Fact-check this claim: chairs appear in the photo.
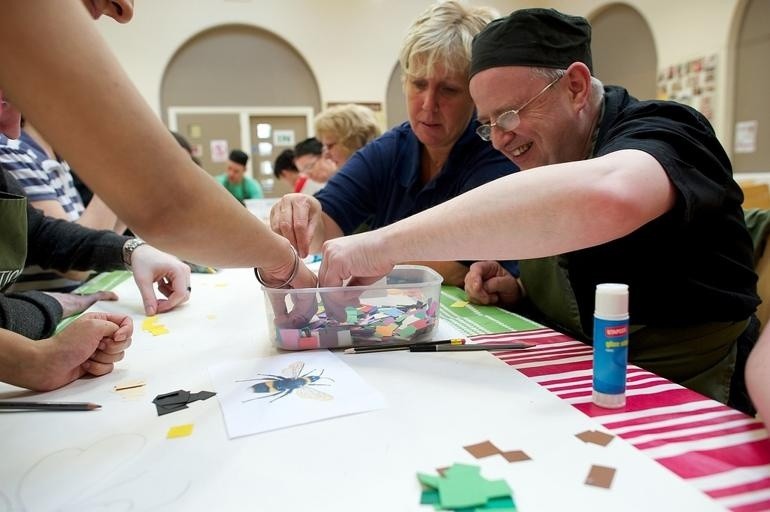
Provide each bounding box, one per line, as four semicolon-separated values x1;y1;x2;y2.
741;208;766;334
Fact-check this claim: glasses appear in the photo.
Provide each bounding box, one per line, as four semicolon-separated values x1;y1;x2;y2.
475;75;562;142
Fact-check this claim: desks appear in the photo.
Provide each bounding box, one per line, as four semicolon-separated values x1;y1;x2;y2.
0;200;770;512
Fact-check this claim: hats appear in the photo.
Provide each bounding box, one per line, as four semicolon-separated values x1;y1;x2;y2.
229;151;249;165
467;8;594;86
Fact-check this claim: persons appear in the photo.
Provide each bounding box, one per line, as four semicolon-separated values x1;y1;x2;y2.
1;1;320;392
1;99;202;340
273;103;387;196
317;7;761;420
213;149;265;200
745;320;770;432
270;1;522;286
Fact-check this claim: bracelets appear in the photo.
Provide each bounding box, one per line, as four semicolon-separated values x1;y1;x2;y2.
254;246;299;287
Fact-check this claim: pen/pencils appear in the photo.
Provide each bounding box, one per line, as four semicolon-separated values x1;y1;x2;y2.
343;339;466;353
409;343;536;352
0;401;103;410
